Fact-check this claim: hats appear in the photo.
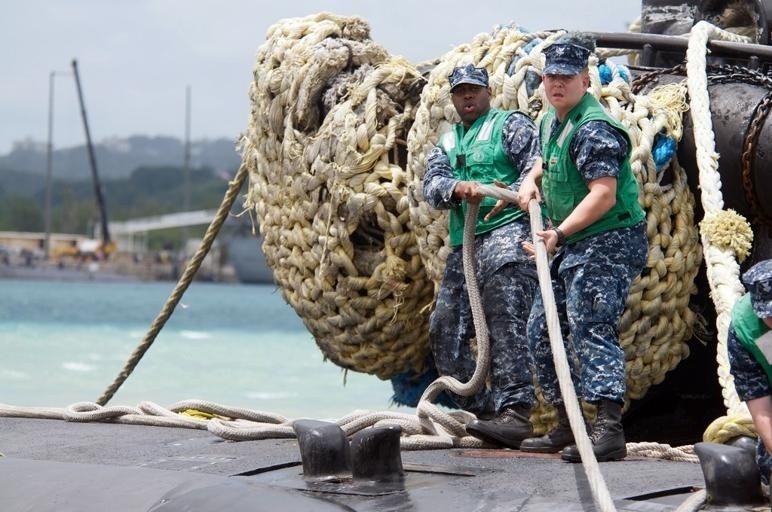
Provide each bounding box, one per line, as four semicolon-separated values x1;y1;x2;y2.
541;43;589;76
448;66;488;92
743;259;771;319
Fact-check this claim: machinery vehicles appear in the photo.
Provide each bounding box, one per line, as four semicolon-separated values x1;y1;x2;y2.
51;57;120;268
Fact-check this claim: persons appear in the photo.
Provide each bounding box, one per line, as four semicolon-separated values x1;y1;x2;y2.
727;258;771;490
518;39;652;463
421;62;543;450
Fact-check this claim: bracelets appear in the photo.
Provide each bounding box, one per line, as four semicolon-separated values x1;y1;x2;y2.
553;227;565;249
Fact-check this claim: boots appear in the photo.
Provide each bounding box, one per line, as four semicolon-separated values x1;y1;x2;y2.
466;407;532;449
521;401;592;453
562;399;627;460
